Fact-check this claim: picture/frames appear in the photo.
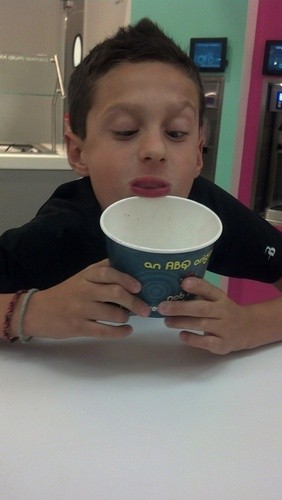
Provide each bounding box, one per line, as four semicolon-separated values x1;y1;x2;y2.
190;37;227;72
262;37;282;76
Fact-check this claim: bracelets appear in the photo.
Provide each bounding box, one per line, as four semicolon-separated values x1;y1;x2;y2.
3;287;37;344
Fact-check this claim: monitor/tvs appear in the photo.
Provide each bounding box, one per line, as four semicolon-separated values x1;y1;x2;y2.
262;40;282;75
190;37;228;72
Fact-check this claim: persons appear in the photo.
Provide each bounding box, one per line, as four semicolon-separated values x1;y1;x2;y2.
0;17;282;355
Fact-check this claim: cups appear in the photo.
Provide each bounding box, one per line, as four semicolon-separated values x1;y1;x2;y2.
100;195;223;319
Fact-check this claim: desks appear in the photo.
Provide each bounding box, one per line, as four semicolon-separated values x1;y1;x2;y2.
0;315;282;500
